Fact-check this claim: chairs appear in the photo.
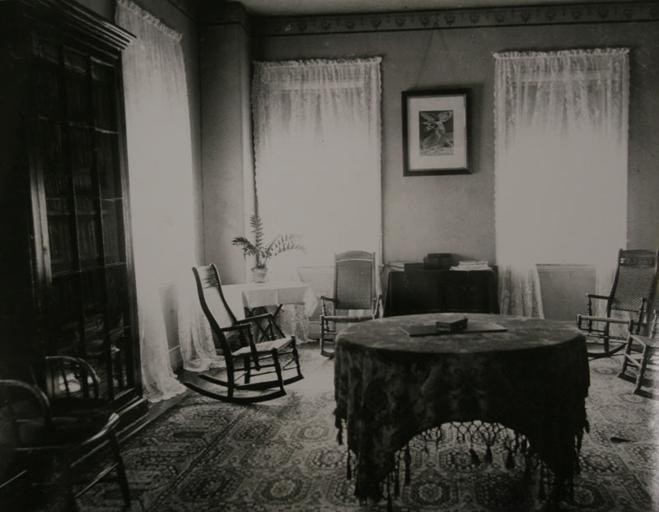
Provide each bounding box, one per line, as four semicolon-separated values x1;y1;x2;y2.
576;249;659;362
1;349;131;511
192;263;304;401
320;250;382;357
617;309;659;393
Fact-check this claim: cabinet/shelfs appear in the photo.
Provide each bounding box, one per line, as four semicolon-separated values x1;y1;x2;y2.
0;0;150;467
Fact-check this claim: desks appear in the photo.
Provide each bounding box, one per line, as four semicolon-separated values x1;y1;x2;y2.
383;264;500;314
203;282;310;344
334;312;591;512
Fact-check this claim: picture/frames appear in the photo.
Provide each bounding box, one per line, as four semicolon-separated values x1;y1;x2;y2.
401;88;472;176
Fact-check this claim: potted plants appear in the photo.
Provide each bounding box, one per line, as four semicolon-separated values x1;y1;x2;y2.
230;215;304;283
